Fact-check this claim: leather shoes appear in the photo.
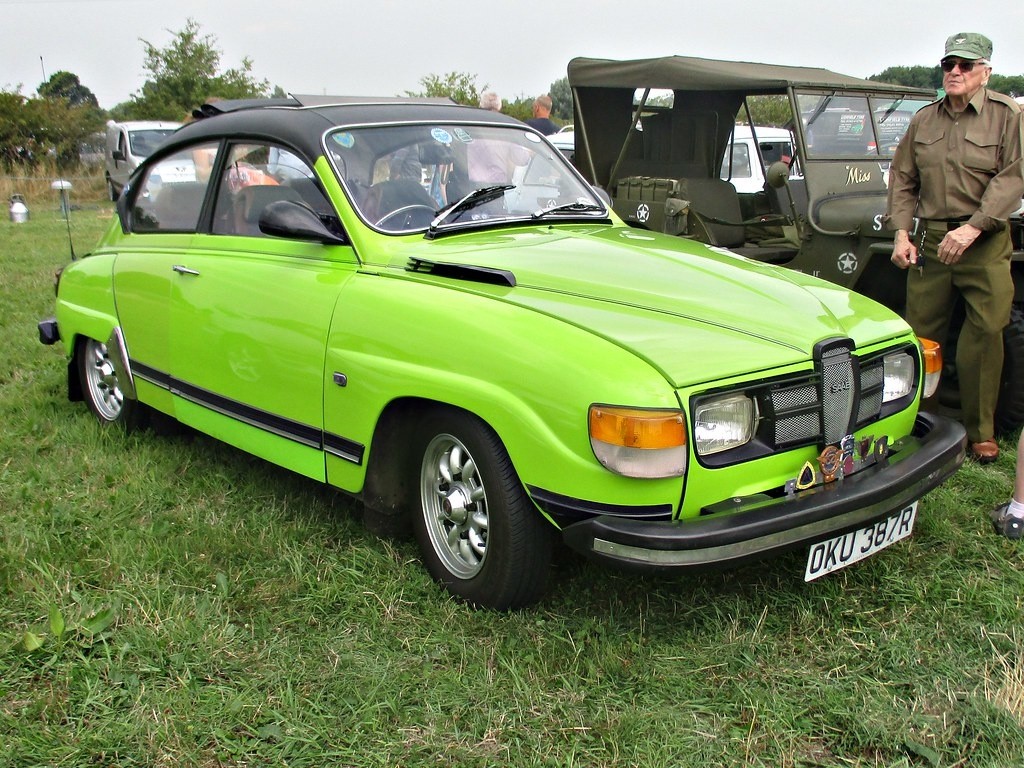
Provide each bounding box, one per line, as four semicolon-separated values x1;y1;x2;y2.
971;435;999;463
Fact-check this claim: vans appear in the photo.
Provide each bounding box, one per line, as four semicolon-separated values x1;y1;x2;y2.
718;124;801;193
104;122;199;207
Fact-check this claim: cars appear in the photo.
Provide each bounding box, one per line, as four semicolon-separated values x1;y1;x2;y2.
546;124;575;151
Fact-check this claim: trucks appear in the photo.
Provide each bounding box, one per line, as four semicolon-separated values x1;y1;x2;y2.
867;101;933;156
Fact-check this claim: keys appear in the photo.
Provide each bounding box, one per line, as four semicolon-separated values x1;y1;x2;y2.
916;255;924;278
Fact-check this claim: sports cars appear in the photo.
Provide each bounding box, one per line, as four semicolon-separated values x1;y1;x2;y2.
39;95;969;614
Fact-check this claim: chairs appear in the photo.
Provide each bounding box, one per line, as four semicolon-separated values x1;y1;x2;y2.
153;168;444;240
679;175;807;263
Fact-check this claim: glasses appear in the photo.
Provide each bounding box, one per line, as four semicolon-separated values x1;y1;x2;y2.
941;61;986;73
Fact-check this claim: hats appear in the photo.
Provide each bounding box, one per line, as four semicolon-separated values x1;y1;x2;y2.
940;33;993;61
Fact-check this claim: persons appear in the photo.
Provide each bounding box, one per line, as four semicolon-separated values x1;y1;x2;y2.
522;97;560;136
267;146;316;184
390;140;421;186
886;31;1024;462
467;89;529;214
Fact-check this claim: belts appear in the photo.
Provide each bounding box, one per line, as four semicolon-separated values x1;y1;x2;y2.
920;217;969;232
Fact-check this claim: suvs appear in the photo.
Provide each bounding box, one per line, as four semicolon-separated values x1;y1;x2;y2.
564;54;1024;438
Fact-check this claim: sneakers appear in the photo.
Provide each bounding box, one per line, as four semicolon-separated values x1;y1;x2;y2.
990;503;1023;540
